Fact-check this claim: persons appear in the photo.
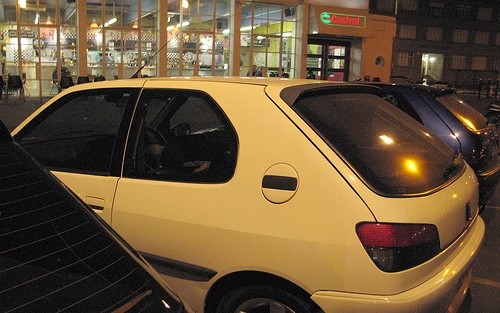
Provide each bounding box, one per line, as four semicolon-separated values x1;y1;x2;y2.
247;65;256;76
307;71;315;79
53;65;57;82
254;65;264;76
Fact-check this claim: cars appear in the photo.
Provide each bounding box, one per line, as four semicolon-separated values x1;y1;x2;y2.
0;120;185;313
9;76;485;313
356;80;499;186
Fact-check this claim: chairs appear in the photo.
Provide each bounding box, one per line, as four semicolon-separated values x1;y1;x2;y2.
61;77;73;88
78;76;92;84
6;76;25;102
